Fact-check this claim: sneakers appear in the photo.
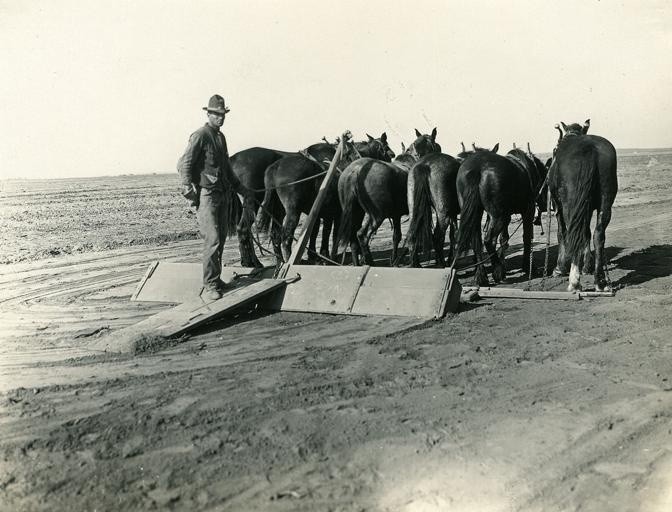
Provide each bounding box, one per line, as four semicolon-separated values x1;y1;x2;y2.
203;279;227;300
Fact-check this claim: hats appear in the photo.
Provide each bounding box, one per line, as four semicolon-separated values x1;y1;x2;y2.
203;95;230;114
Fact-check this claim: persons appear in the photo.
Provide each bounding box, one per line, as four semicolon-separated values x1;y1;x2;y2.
177;94;247;300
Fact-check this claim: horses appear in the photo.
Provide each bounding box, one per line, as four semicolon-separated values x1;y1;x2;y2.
454;143;554;286
220;137;351;267
338;128;441;266
391;143;501;267
254;132;395;265
547;119;619;293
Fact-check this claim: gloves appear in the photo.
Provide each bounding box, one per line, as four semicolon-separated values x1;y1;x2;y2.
183;190;199;214
